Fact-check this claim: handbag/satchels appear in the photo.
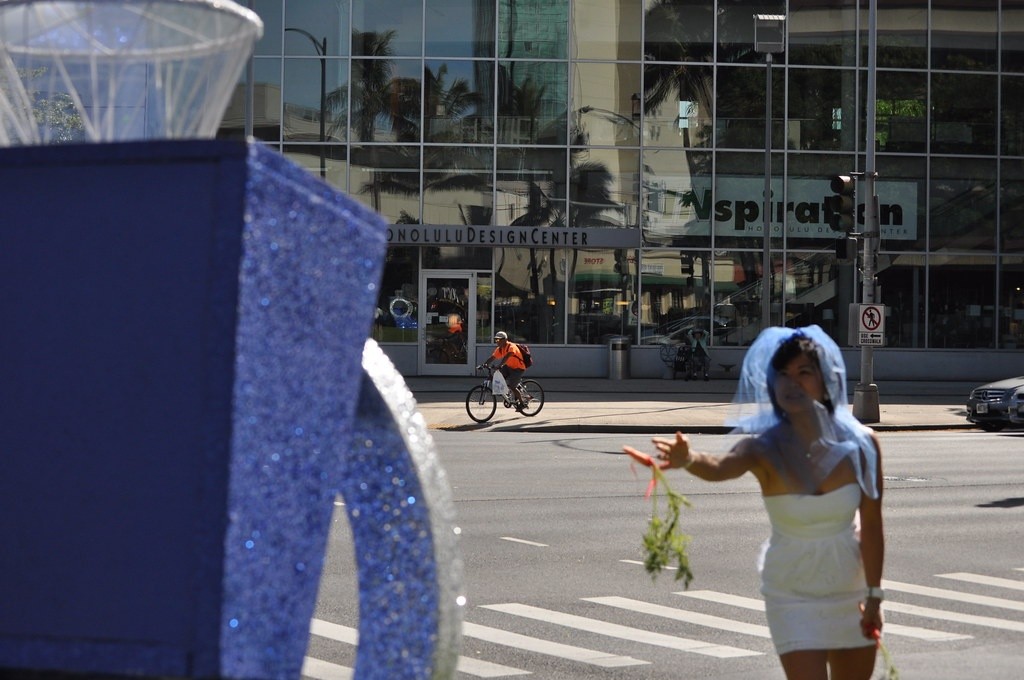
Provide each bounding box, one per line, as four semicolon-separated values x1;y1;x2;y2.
492;369;508;394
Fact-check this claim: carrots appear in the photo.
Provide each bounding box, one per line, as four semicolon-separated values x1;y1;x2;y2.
858;599;886;641
621;445;656;467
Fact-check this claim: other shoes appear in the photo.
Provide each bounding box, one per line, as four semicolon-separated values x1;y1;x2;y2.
693;376;697;380
515;402;526;412
705;376;709;381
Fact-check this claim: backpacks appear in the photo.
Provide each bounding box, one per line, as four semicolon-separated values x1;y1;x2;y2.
499;343;533;368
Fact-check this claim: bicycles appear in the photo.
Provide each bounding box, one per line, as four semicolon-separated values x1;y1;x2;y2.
466;362;544;423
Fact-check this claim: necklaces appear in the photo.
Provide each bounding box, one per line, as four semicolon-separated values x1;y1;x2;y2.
795;439;819;459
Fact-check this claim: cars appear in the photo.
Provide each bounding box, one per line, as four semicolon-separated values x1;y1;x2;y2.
966;375;1024;434
639;314;733;344
427;298;466;325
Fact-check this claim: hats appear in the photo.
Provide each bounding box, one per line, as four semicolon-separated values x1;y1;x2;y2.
493;332;508;340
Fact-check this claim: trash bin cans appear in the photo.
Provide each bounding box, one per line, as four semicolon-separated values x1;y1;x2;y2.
608;337;631;379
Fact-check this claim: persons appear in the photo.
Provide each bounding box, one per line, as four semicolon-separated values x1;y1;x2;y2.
446;306;465;356
652;325;885;680
482;332;527;412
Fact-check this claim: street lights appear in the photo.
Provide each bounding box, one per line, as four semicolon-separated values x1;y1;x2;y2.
284;27;328;180
755;13;787;328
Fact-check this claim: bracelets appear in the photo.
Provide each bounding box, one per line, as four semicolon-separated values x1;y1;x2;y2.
865;587;884;601
685;454;695;470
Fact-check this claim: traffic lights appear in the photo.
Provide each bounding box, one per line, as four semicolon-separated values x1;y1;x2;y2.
829;174;856;232
